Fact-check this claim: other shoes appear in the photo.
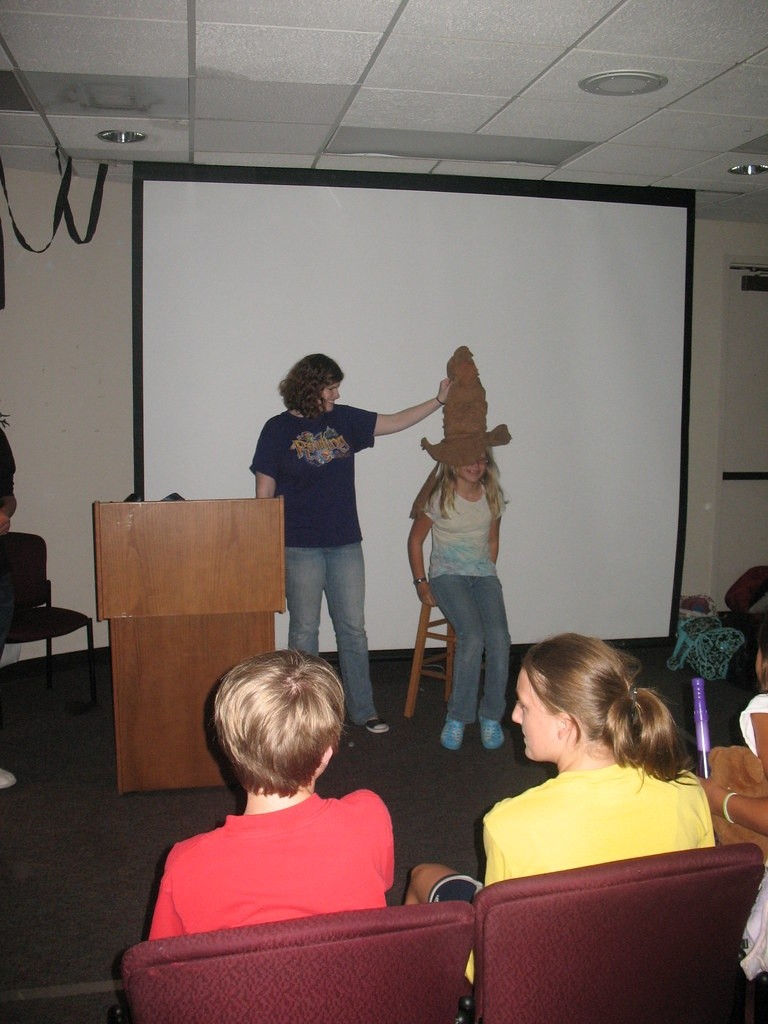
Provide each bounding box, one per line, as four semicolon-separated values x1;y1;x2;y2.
364;716;390;733
440;713;466;751
479;709;506;750
0;768;17;789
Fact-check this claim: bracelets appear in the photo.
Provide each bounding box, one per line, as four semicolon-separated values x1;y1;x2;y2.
413;578;426;585
723;793;737;823
437;397;446;405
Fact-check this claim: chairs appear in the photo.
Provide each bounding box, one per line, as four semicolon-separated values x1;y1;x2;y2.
106;900;471;1024
471;843;766;1024
0;533;96;706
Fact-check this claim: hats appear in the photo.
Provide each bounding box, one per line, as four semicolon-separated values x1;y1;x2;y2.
409;346;512;519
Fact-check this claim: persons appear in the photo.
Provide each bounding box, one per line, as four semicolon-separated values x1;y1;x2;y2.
404;632;715;984
249;354;454;734
148;651;395;940
0;428;17;789
700;614;768;866
407;447;511;750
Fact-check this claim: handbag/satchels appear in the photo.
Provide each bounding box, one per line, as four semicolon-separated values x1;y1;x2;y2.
667;616;744;680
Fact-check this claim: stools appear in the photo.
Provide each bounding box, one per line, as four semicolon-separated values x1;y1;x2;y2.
405;604;486;718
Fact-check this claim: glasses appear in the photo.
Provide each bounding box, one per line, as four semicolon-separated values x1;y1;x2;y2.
477;457;490;466
